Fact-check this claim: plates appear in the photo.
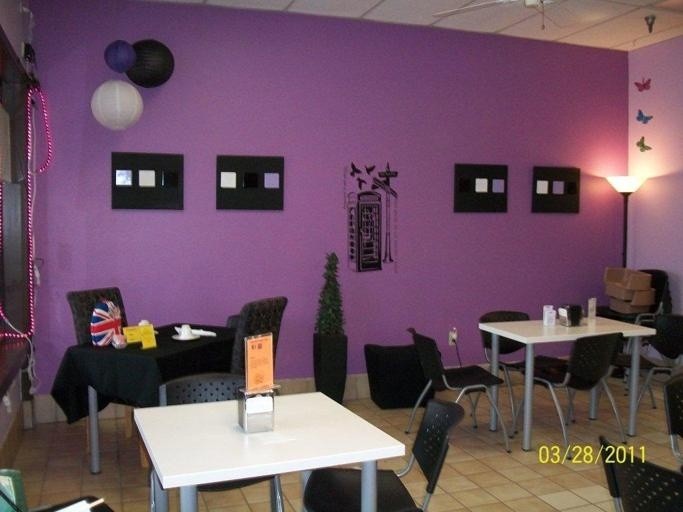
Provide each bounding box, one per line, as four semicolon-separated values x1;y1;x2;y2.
171;335;201;340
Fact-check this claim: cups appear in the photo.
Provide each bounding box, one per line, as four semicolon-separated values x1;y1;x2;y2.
138;319;149;326
179;324;192;337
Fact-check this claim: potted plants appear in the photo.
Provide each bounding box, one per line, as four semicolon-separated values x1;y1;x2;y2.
314;253;348;404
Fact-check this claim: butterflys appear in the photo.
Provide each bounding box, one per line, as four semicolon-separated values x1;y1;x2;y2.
636;136;652;152
634;77;652;92
636;109;653;124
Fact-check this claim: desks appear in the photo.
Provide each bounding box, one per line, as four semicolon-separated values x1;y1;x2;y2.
134;392;405;512
51;323;237;475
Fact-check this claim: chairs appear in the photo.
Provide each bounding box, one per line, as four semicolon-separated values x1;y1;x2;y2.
231;296;288;376
159;373;284;512
66;287;151;469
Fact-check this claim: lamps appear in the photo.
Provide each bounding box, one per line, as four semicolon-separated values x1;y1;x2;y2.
606;175;647;269
